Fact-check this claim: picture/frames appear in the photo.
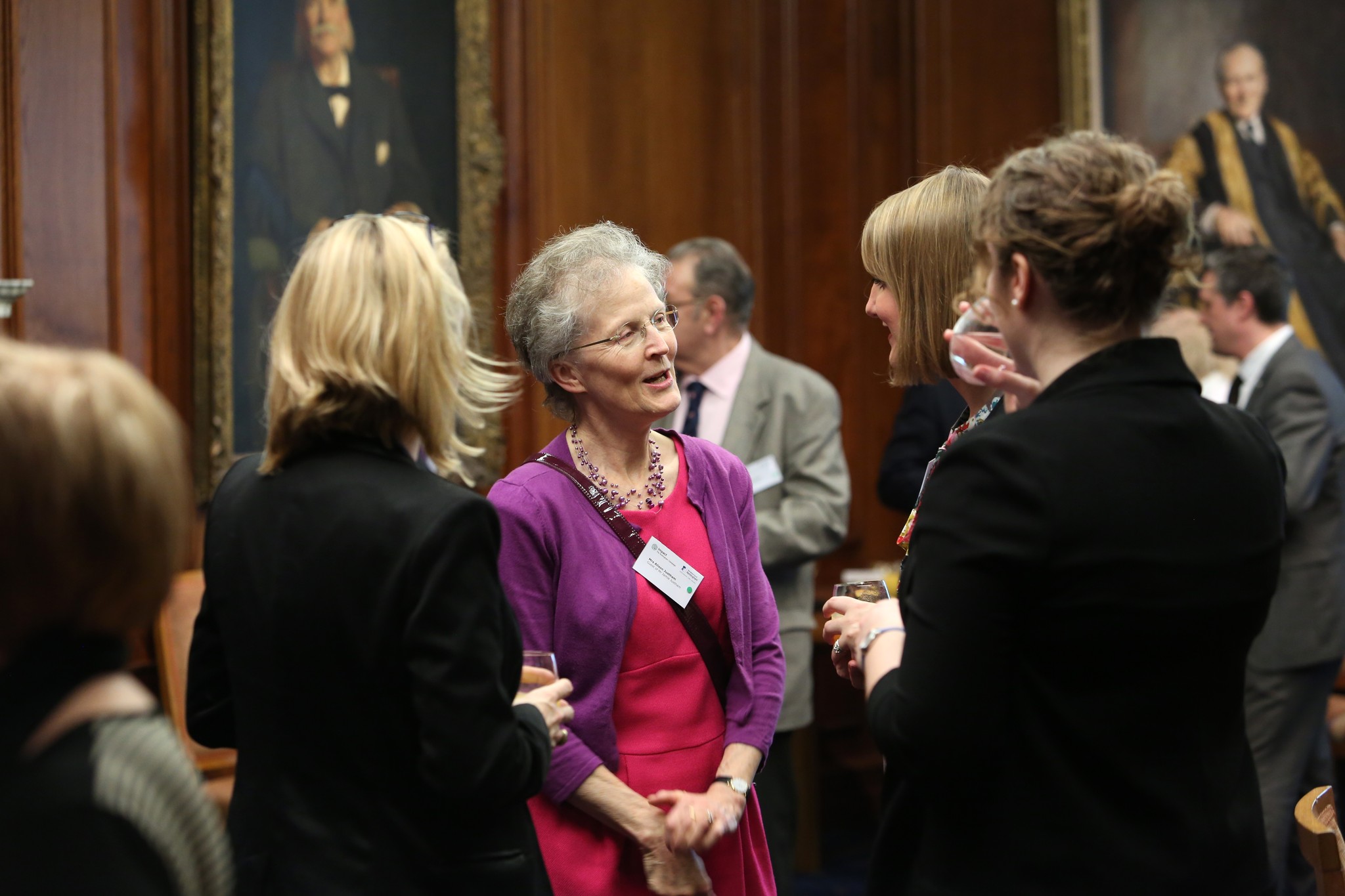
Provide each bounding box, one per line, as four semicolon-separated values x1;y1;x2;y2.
186;0;498;509
1058;0;1345;314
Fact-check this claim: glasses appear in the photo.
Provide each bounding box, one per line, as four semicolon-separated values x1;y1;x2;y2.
553;304;680;359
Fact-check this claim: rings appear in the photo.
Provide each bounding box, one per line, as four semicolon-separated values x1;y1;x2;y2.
560;728;568;745
705;808;714;825
688;805;698;824
833;639;846;654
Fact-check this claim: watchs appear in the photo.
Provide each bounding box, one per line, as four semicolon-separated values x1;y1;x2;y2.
712;775;750;800
860;626;905;673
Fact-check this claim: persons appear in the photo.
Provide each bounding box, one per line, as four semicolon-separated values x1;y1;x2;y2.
1198;245;1345;896
486;221;785;896
184;212;574;895
1160;42;1345;383
0;343;235;896
821;130;1288;896
665;238;851;896
254;1;429;295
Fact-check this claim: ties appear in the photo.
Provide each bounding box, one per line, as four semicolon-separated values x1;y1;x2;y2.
683;383;707;437
1227;375;1243;409
1246;125;1259;144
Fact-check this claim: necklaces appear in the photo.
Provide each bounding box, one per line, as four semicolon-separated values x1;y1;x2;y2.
569;421;665;514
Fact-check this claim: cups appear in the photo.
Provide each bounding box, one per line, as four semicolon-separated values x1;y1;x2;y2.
512;649;560;696
949;297;1016;386
831;580;890;646
